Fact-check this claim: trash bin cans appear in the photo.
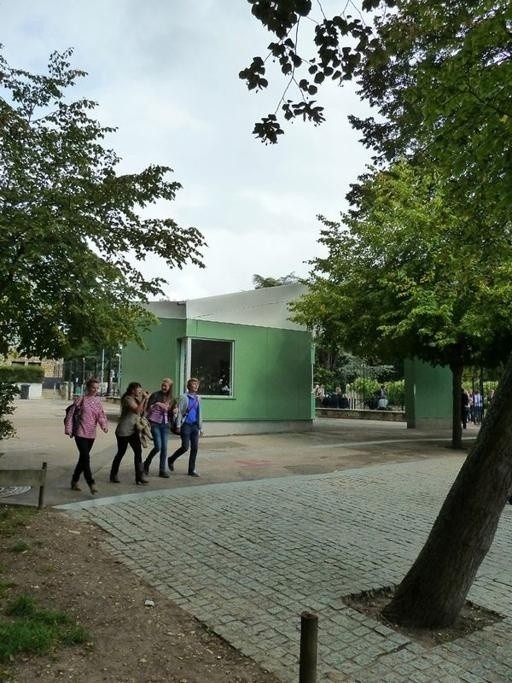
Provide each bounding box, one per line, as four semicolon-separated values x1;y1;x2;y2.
21;385;30;398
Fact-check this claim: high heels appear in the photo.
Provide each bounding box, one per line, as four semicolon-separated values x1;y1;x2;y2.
71;481;81;490
90;484;98;495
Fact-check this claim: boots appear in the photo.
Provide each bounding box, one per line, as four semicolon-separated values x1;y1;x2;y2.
109;469;120;483
135;471;149;485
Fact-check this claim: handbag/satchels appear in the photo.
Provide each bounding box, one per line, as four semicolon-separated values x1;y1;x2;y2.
170;422;181;435
64;402;84;438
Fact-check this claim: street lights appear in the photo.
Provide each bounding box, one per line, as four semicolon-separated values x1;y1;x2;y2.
115;352;121;387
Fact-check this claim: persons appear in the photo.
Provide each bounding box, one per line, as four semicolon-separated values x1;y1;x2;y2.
460;384;496;429
312;383;389;411
109;380;152;485
166;377;206;478
142;376;178;477
64;378;112;496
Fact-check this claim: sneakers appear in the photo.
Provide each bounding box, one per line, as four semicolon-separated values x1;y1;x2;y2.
168;457;174;471
144;464;150;475
188;470;200;477
159;472;170;478
